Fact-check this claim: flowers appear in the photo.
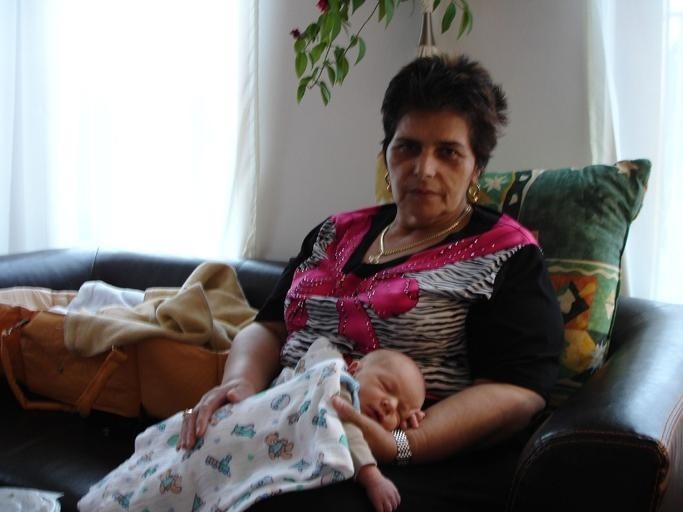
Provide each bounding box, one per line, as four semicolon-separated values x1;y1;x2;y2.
290;0;472;107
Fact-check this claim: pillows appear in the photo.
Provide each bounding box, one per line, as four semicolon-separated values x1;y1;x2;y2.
376;151;650;411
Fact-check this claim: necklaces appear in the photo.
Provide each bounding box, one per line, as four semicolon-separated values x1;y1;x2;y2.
367;204;472;267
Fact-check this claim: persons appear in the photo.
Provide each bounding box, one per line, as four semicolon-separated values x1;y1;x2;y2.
74;348;429;511
176;54;566;512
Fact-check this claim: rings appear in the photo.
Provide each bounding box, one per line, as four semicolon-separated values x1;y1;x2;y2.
182;407;193;417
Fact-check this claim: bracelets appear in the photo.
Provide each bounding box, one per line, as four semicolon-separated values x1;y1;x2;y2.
392;428;413;469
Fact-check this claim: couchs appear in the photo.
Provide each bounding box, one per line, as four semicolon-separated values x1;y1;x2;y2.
1;247;682;512
